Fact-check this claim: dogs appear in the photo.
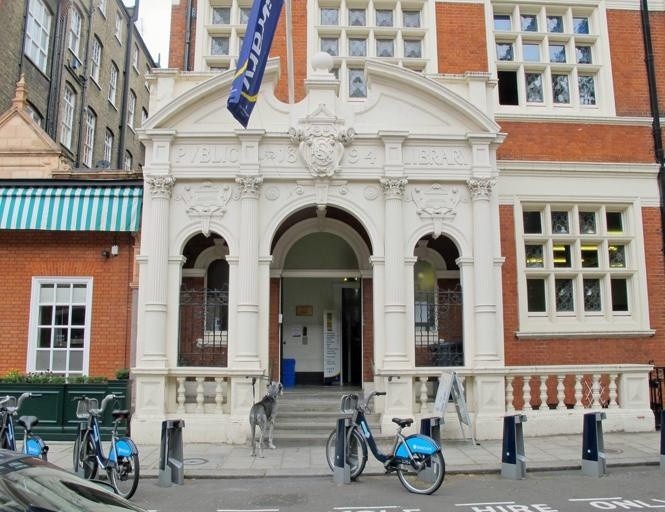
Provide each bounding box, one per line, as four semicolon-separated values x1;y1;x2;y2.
248;382;284;458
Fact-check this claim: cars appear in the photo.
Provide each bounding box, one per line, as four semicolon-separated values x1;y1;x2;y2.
0;446;143;512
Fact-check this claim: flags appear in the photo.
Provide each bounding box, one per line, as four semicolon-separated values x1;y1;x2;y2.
226;1;283;126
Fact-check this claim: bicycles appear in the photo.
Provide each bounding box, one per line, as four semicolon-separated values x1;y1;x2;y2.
0;391;141;500
326;390;446;494
